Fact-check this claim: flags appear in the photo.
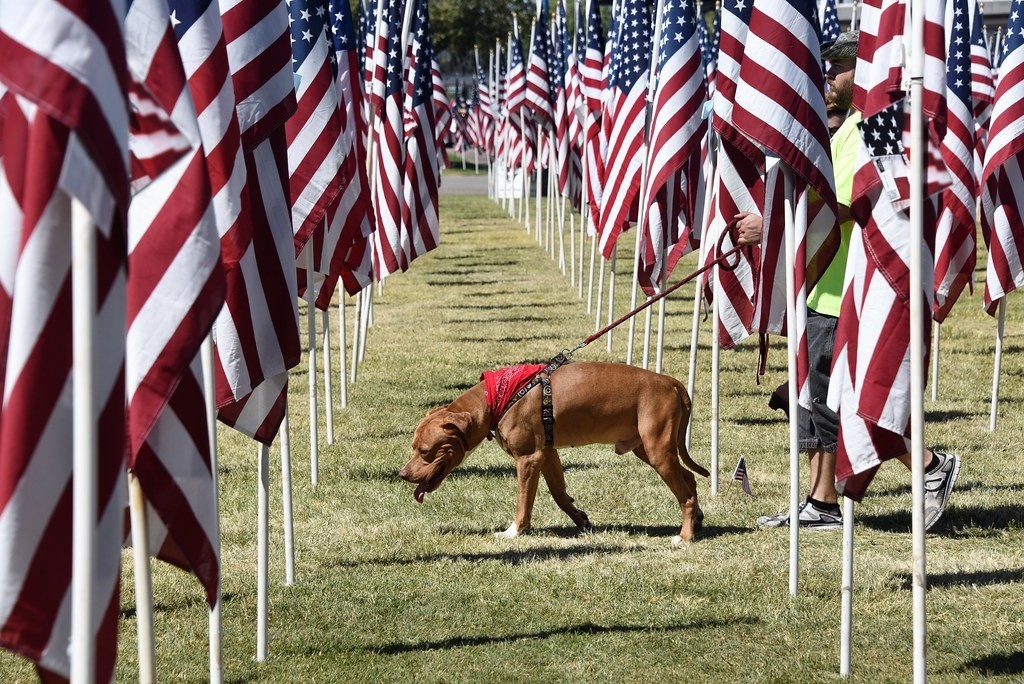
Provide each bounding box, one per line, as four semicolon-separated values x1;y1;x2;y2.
0;0;1024;684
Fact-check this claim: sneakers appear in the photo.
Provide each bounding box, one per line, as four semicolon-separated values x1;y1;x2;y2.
757;495;843;530
925;450;961;530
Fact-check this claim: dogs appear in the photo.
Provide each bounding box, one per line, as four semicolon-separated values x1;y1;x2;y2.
398;362;710;544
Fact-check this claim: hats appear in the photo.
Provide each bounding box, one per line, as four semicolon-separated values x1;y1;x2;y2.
820;30;859;58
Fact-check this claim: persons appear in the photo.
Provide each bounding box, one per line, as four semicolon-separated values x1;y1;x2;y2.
733;32;961;531
769;86;849;421
